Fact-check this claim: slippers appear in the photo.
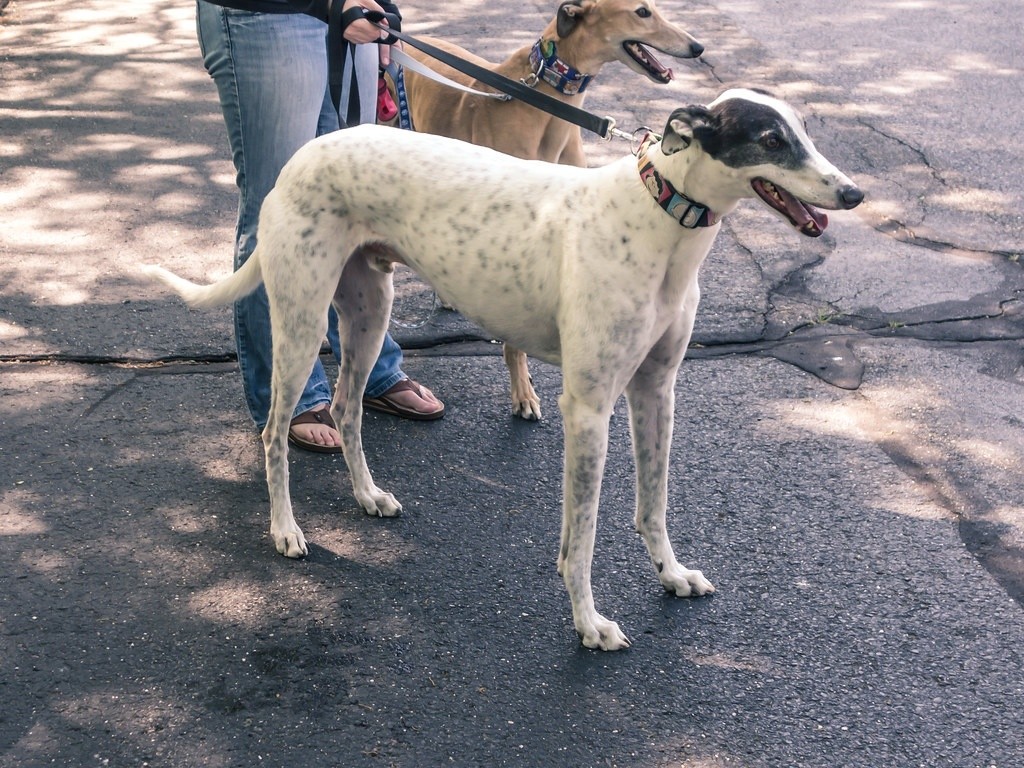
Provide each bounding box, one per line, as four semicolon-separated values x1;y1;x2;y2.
333;376;447;421
290;402;343;452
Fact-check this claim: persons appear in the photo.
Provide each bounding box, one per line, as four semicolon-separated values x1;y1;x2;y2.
196;0;444;453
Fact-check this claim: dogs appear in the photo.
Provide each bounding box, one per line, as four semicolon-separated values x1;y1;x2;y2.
376;1;706;424
136;84;865;652
